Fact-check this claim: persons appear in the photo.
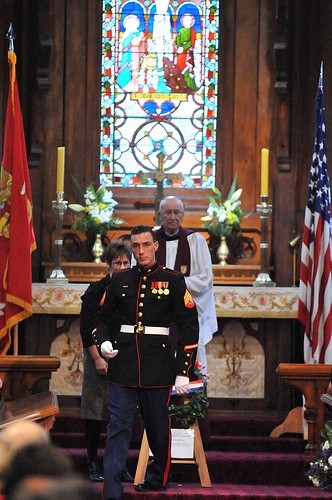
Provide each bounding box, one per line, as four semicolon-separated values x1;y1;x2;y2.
0;196;218;500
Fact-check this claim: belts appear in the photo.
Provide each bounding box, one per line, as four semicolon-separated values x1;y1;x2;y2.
120;325;169;336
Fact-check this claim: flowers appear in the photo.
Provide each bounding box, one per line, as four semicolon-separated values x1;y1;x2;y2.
194;359;211;384
200;171;243;236
67;181;125;233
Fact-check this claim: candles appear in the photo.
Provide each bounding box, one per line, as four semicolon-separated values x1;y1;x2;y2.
56;146;65;192
261;148;269;196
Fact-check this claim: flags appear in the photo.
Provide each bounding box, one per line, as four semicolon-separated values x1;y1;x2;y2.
297;62;332;440
0;51;36;388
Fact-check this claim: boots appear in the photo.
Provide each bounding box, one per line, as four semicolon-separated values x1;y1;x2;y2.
87;453;105;482
120;468;134;482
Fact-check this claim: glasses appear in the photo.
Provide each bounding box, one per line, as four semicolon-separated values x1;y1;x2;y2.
110;261;130;268
163;210;182;216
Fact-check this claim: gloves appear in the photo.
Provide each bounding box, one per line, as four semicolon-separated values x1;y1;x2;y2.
175;376;191;395
100;340;118;359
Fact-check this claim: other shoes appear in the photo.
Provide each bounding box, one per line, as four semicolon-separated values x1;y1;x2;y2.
135;480;161;491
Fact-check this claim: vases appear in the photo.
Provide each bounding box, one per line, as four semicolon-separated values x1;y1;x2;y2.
216;236;229;265
92;234;105;263
